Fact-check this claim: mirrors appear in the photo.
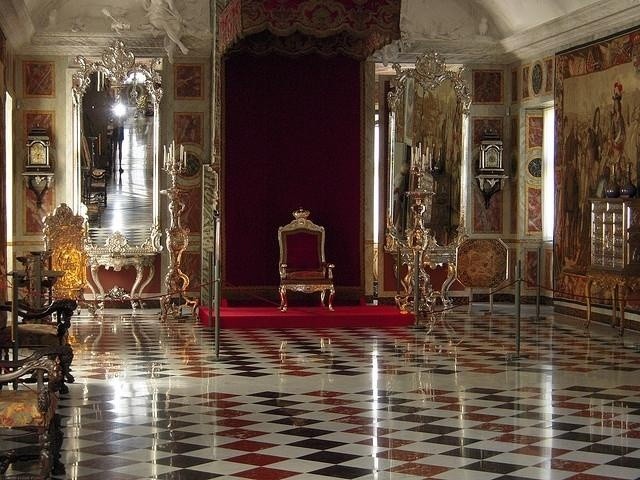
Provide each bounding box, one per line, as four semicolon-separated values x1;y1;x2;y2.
71;35;164;312
383;47;474;314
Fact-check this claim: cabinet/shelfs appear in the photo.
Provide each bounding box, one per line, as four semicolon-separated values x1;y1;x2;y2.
588;196;639;273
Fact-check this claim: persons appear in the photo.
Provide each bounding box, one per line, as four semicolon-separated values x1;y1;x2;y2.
563;90;626;221
113;108;126;173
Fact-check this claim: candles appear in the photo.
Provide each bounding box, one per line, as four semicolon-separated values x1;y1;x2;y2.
409;141;435;173
161;140;188;170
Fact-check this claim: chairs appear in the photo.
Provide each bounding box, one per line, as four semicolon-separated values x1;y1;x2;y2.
1;296;75;480
277;208;338;313
13;202;85;318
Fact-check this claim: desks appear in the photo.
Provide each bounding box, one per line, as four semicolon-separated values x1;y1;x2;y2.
562;266;640;345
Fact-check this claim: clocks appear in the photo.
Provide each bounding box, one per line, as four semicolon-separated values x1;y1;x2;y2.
20;123;56;210
474;127;510;209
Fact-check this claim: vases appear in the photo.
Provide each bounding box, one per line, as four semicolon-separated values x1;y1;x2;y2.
606;160;637;198
